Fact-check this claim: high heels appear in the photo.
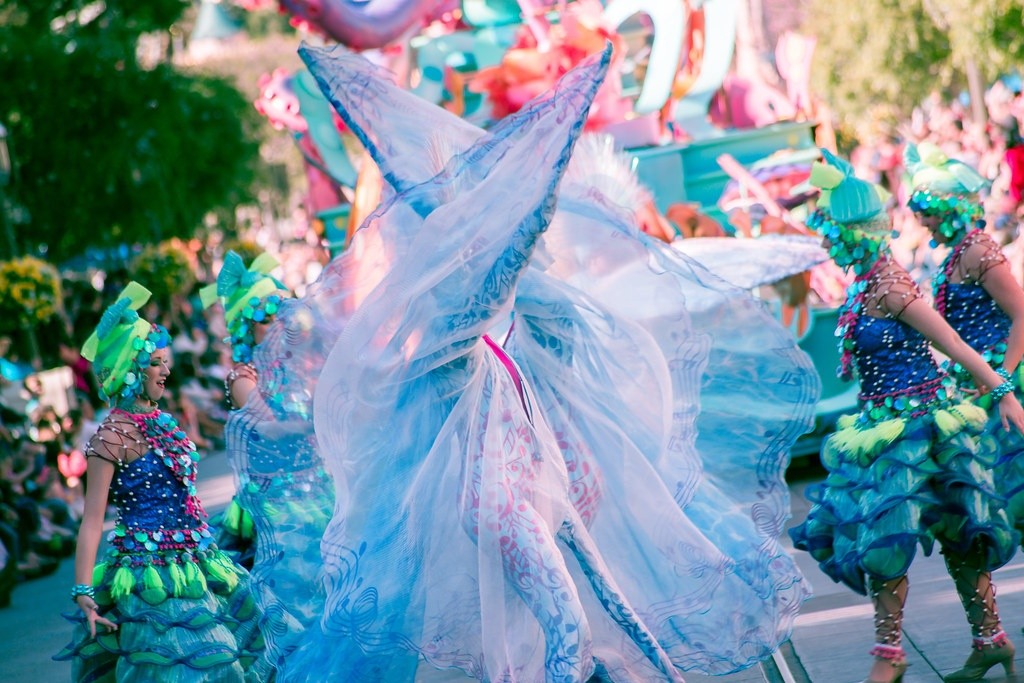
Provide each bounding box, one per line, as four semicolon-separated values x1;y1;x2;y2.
857;655;912;683
943;638;1015;683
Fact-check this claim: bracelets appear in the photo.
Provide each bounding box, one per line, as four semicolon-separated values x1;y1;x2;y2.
993;367;1014;383
70;584;96;602
991;381;1016;403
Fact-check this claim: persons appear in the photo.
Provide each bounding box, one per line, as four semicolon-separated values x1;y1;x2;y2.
0;74;1024;683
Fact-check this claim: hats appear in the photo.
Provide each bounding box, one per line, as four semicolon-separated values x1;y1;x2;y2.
809;148;892;223
201;249;289;323
81;281;153;396
906;142;992;194
